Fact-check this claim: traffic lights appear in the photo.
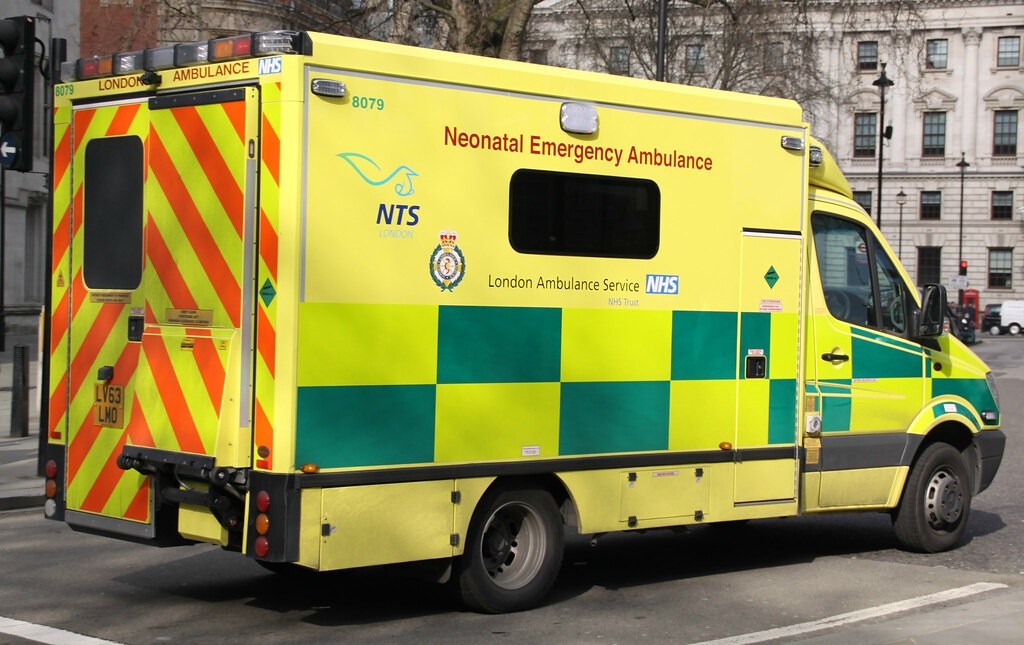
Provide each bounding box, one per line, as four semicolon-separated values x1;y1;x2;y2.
959;260;968;276
0;13;39;173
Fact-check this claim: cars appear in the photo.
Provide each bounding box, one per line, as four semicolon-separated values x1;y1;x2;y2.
979;298;1010;336
999;300;1024;336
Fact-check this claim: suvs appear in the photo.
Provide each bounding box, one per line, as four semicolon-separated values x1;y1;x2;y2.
868;282;978;345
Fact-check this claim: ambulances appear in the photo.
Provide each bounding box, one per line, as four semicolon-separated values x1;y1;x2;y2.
36;26;1012;618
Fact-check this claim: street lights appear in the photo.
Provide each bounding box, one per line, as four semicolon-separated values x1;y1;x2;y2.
896;186;907;262
869;60;895;229
954;151;972;307
